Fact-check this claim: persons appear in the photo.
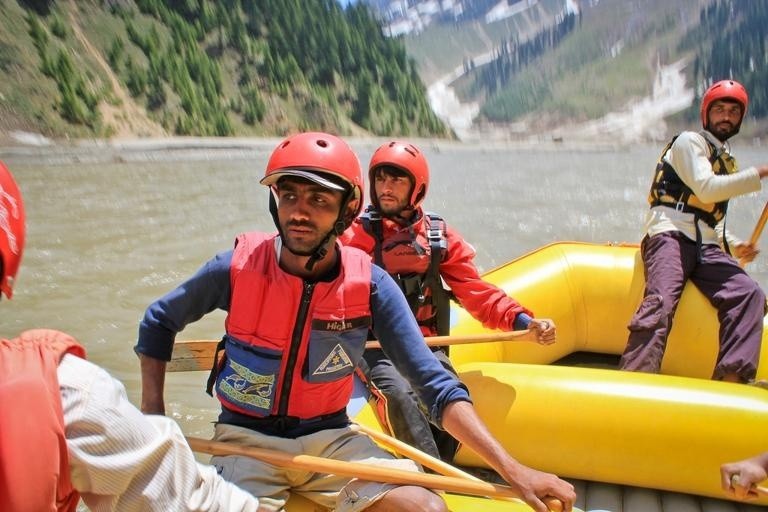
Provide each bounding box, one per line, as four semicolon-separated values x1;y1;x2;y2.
620;78;768;390
1;158;262;511
719;451;768;500
335;138;556;494
131;129;580;512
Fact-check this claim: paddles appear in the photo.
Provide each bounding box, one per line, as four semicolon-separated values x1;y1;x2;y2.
165;328;543;372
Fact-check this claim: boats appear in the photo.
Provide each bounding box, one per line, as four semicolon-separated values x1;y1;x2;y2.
271;237;768;505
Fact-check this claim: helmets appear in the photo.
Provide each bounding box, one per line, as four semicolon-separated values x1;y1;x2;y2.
702;80;749;134
260;131;364;231
0;160;25;299
368;141;429;212
